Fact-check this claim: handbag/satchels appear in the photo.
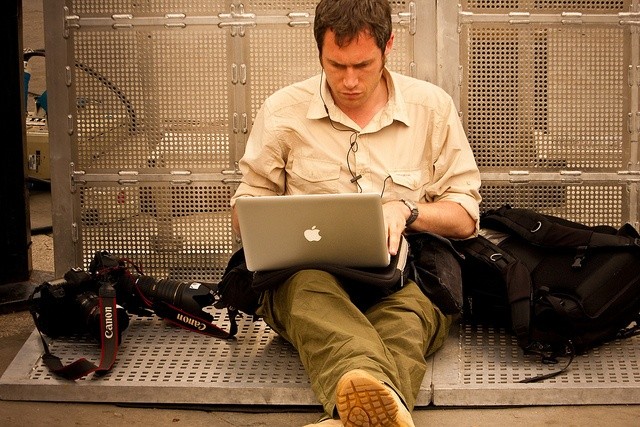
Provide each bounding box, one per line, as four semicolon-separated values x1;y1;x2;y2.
250;234;412;304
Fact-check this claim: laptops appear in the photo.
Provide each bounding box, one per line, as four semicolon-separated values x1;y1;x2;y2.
235;193;390;272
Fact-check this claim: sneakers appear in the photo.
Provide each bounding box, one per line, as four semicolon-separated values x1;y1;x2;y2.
336;369;415;425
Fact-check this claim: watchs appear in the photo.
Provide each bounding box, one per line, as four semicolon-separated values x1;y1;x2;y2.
399;198;418;226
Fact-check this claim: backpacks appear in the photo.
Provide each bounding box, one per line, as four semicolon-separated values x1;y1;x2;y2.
456;206;640;384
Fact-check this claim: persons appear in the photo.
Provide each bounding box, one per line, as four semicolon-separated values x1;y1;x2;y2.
229;0;483;426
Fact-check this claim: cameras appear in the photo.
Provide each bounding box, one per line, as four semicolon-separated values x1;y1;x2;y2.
29;267;129;346
90;250;218;324
